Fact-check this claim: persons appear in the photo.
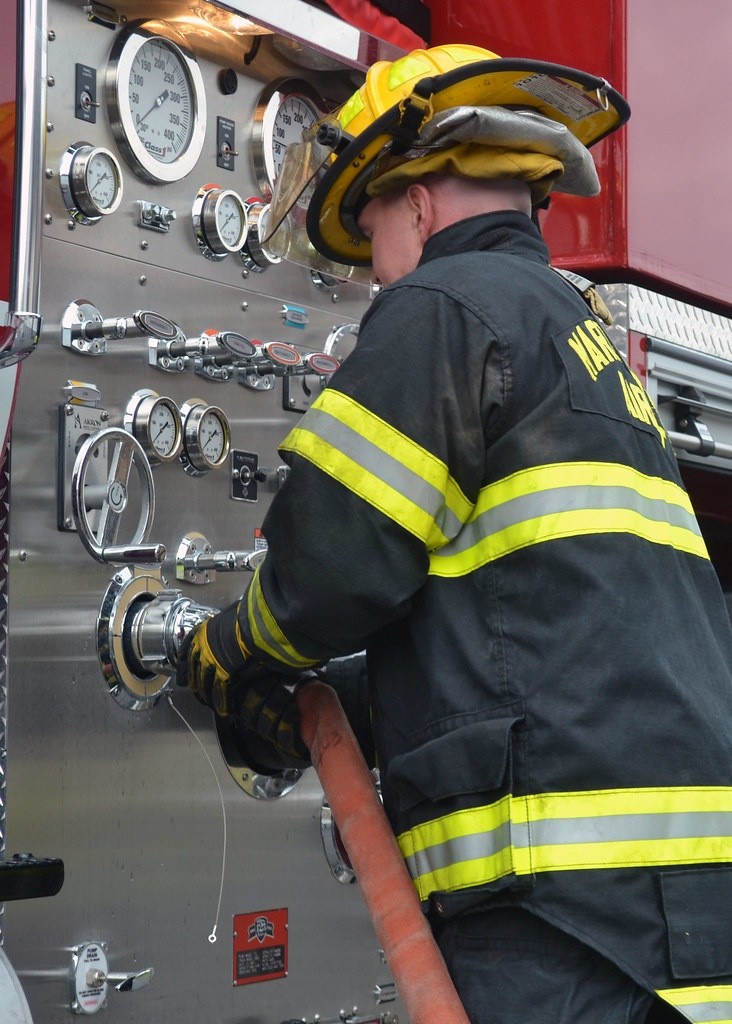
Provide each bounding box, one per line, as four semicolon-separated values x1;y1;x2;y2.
178;44;732;1024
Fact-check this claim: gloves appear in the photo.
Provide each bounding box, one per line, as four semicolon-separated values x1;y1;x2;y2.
177;601;266;715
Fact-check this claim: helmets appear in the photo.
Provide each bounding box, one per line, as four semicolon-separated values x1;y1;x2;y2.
307;46;631;267
